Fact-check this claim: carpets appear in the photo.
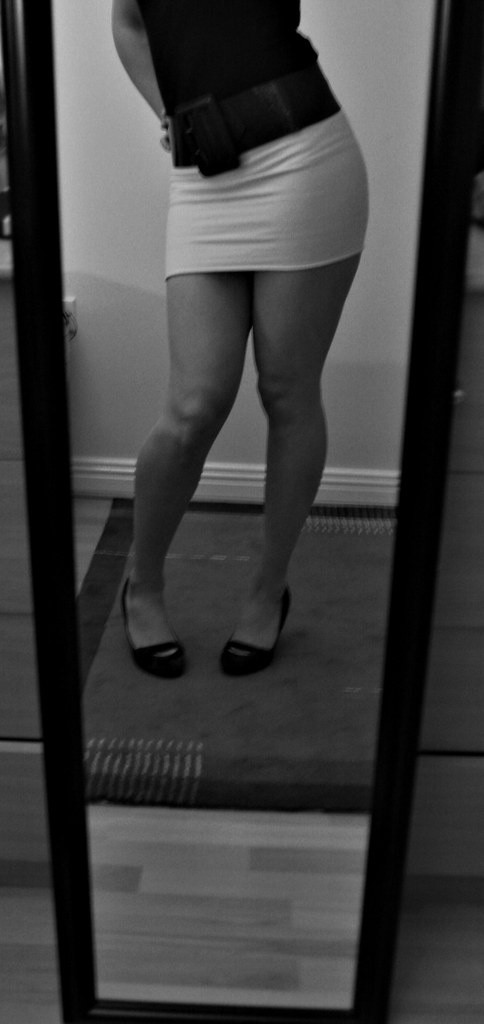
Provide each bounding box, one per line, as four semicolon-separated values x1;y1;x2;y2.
76;497;399;815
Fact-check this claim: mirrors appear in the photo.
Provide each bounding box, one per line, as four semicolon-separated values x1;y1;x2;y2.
1;1;484;1024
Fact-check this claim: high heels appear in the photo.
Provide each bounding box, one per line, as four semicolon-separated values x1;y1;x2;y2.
121;578;186;679
220;583;290;676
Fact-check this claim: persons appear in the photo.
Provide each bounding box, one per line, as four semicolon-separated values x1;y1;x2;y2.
111;1;369;678
